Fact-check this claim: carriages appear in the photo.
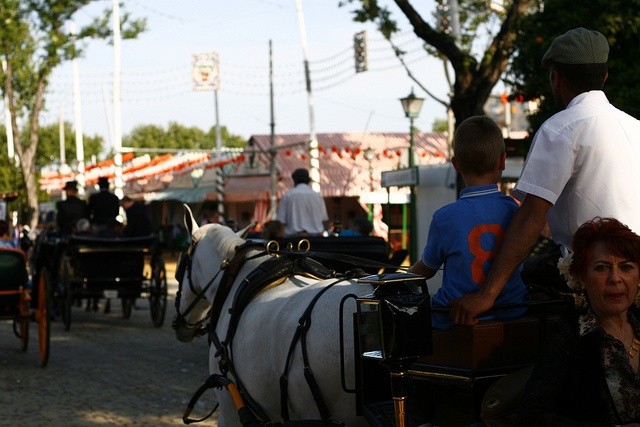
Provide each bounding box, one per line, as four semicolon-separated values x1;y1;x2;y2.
171;202;640;427
15;217;173;331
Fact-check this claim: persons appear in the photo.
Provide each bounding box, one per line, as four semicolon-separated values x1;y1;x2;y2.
119;196;155;236
406;115;527;331
0;219;16;248
55;180;91;229
86;177;124;236
275;168;329;238
519;216;640;427
446;27;640;327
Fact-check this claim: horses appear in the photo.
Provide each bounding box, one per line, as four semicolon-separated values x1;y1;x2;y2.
172;202;378;427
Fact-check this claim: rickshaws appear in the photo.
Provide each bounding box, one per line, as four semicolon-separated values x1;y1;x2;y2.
0;243;52;369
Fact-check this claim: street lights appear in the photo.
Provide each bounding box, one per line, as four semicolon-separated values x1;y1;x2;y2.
400;86;426;267
364;147;376;237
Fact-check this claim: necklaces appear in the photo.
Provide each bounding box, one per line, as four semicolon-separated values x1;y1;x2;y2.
605;324;639;360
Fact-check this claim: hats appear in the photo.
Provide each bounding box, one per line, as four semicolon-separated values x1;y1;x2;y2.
96;177;110;185
292;168;314;186
62;181;78;191
537;28;611;68
